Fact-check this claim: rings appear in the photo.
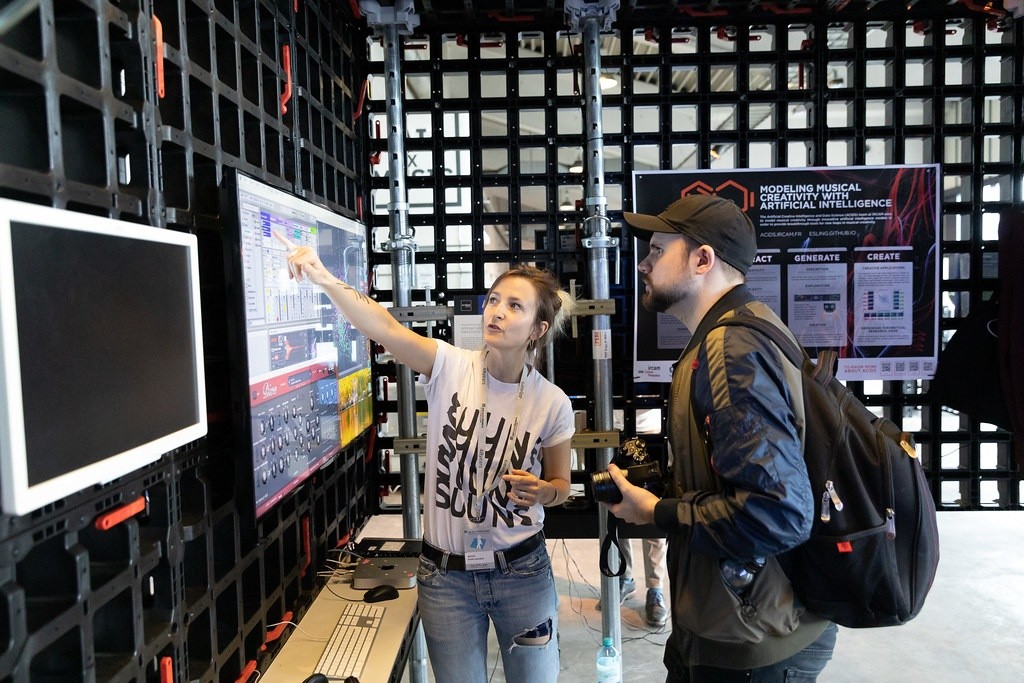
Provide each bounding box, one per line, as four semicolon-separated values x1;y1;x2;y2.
518;492;526;497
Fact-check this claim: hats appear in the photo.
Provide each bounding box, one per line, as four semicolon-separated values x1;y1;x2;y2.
623;196;756;275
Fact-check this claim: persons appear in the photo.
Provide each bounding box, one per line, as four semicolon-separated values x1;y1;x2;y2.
603;194;838;683
274;230;575;683
599;538;668;626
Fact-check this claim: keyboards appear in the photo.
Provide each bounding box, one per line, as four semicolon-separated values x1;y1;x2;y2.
311;603;386;681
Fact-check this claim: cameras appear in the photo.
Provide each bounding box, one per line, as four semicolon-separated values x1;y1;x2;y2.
590;436;674;503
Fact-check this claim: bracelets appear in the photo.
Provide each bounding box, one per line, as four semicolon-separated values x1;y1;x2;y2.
543;489;559;506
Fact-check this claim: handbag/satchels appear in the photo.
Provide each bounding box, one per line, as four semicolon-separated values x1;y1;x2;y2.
927;287;1016;431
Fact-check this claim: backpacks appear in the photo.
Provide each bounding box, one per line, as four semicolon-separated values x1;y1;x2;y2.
690;316;940;628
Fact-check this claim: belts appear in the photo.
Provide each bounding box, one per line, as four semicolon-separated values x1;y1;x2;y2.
421;529;544;571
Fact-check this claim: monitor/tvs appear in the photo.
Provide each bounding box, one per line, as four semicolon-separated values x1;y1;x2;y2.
0;196;210;517
201;166;378;527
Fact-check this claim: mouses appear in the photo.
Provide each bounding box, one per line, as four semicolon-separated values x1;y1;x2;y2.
363;584;400;603
